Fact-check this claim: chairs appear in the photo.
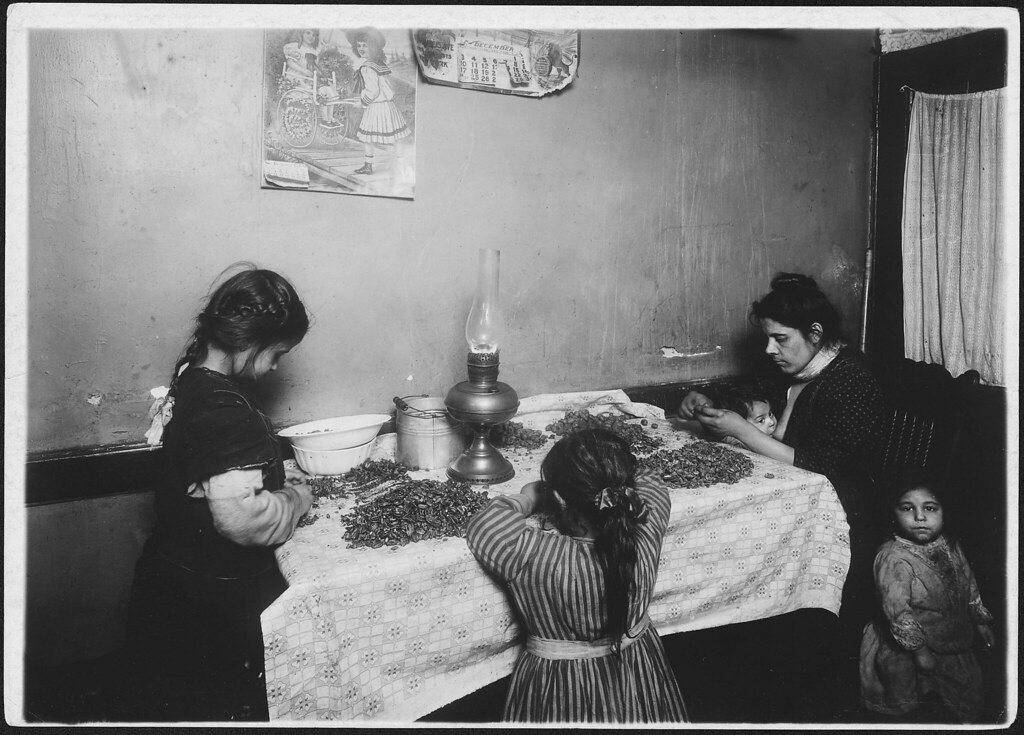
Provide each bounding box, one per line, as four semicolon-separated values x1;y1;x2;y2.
801;350;982;729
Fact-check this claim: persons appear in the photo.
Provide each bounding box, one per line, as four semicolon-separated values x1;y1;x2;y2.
722;377;813;450
860;469;998;725
125;269;319;722
679;273;892;585
464;428;690;724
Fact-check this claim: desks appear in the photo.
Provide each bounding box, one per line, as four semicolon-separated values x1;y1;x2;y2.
262;415;852;723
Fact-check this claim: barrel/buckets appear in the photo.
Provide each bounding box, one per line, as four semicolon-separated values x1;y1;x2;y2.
393;394;467;470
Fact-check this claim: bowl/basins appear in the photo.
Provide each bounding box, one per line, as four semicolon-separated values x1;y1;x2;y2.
290;438;378;477
278;413;391;450
